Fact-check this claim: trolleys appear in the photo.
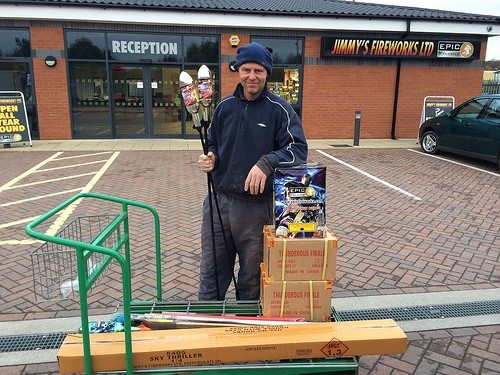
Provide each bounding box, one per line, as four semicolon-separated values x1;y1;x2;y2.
25;191;360;375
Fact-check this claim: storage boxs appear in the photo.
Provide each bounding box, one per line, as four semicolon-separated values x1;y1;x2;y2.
263;225;337;281
259;263;332;321
57;318;407;374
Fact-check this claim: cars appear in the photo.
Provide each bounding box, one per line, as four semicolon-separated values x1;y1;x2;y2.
418;95;500;169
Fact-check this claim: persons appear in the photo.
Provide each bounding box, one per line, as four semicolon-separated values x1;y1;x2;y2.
194;41;310;318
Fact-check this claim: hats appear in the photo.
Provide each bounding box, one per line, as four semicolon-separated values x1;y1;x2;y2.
233;41;273;78
